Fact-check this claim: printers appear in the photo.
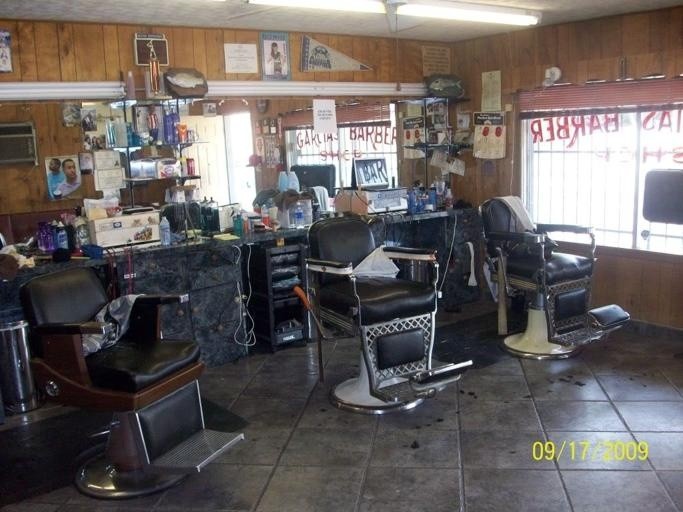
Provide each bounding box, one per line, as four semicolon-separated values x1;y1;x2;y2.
89;203;161;250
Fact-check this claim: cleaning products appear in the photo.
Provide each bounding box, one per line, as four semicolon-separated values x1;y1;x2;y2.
230;208;248;234
445;185;454;209
288;168;300;191
37;220;68;252
410;178;420;191
278;169;288;193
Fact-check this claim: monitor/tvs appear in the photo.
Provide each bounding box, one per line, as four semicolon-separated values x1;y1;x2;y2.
350;158;389;189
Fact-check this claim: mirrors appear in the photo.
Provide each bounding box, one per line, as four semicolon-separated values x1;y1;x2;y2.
0;97;452;254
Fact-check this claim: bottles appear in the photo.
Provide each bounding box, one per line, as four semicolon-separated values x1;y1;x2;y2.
445;188;454;212
158;215;171;246
125;69;136;101
293;201;305;230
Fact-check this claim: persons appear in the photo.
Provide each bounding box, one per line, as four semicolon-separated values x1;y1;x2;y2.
57;159;81;198
266;42;286;74
45;158;65;200
146;40;158;61
146;103;161;145
81;108;107;152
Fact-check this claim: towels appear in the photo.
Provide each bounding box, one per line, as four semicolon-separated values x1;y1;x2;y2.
478;197;538;232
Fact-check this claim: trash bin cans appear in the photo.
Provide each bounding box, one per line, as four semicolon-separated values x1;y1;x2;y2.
0;319;41;416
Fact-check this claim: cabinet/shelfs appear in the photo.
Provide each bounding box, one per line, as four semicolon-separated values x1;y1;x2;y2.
103;98;209;215
243;242;309;352
116;245;248;367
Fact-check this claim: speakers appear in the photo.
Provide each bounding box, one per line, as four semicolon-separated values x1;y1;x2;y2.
0;121;39;163
134;31;170;67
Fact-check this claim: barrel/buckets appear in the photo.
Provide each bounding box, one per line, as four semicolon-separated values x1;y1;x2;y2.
1;309;37;414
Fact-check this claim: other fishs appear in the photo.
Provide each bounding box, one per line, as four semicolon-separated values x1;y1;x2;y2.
167;72;208;89
426;77;461;91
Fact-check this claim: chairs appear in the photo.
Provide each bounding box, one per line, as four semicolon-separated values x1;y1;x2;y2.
19;266;244;500
294;217;474;417
479;196;631;362
